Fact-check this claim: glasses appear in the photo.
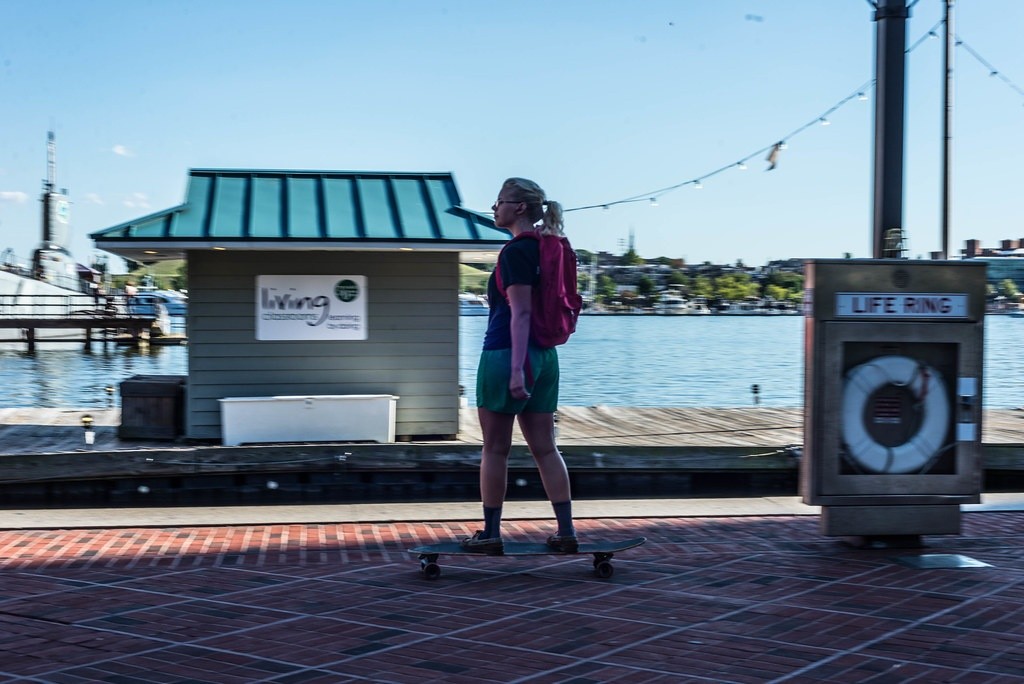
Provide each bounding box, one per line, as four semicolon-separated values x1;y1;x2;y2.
496;200;521;208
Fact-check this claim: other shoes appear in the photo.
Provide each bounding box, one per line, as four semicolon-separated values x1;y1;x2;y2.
546;529;579;554
462;529;504;556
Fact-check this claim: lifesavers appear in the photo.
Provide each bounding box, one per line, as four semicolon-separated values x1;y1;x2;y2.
841;352;954;476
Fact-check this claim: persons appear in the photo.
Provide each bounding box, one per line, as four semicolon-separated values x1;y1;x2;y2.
124;279;139;316
460;178;579;555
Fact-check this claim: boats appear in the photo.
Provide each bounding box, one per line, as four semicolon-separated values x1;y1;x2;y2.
1009;309;1024;318
110;289;189;317
0;130;108;341
578;295;803;315
459;295;491;316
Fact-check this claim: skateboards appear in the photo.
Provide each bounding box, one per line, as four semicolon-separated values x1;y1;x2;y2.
404;534;651;582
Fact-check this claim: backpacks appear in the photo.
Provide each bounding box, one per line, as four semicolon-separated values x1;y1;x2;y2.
496;229;582;348
